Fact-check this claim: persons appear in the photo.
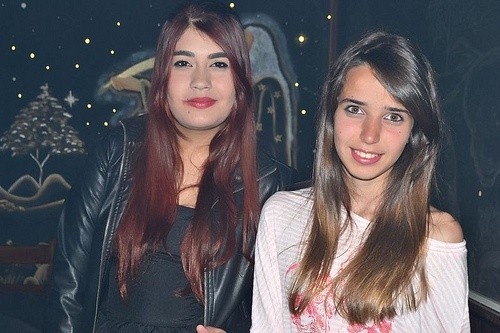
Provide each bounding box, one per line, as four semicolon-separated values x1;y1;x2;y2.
44;0;298;332
247;31;472;333
